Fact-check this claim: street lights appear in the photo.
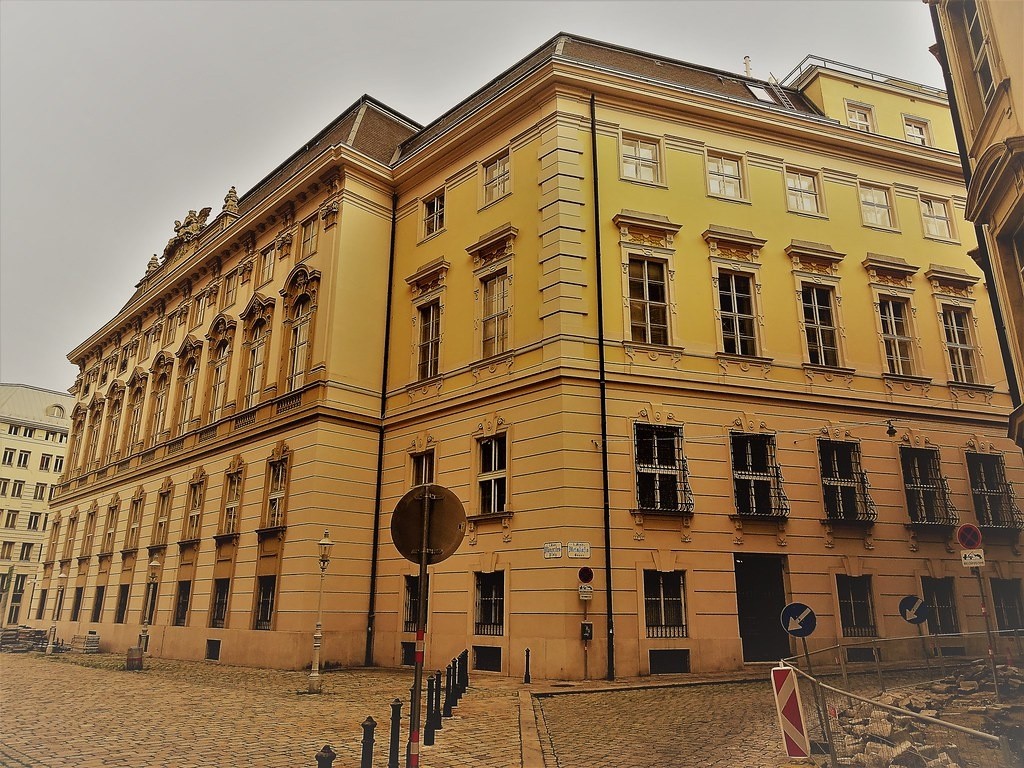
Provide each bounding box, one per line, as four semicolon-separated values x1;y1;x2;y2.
44;569;68;658
308;530;335;693
138;554;161;664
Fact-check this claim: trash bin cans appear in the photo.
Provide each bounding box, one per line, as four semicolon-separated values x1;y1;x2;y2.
126;645;145;671
